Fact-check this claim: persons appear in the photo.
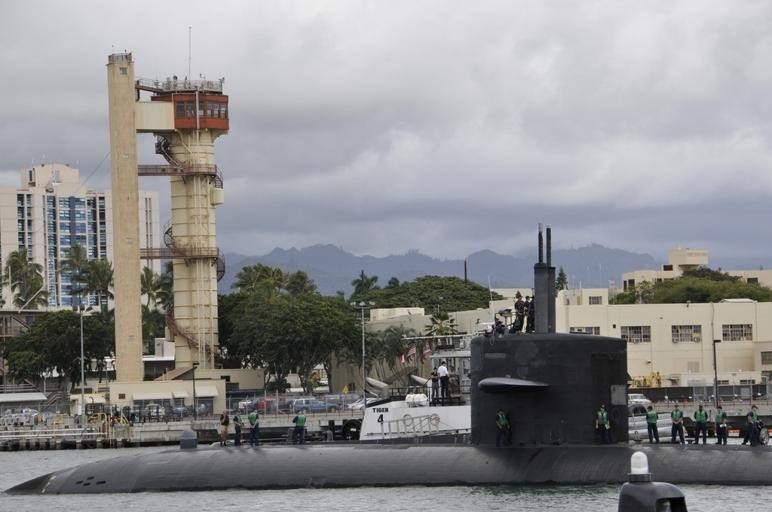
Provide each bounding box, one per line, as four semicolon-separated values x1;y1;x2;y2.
248;407;260;446
693;404;708;445
220;408;228;446
742;404;764;446
233;409;244;445
514;293;535;333
293;409;307;444
437;360;449;398
596;404;611;445
496;408;510;447
671;403;685;445
430;366;440;403
646;405;660;443
714;405;728;445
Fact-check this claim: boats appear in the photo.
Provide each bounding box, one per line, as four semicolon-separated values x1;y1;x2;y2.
359;323;494;441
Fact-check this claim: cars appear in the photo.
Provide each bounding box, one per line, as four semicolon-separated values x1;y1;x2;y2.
348;396;382;410
627;393;652;415
146;403;165;419
241;396;340;413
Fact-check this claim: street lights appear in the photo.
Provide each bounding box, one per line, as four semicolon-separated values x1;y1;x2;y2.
71;301;92;413
713;338;721;407
350;300;376;409
192;361;201;418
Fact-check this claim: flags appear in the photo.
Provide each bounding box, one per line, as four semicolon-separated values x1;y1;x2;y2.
399;340;431;365
